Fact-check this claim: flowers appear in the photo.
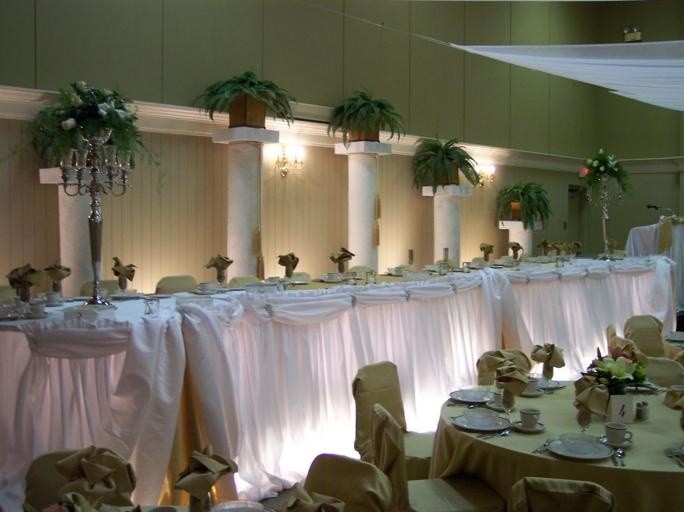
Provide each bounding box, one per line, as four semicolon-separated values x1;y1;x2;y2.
578;147;632;193
581;345;657;396
32;80;140;167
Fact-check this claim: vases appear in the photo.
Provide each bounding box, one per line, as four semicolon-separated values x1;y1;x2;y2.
66;166;113;182
605;393;634;425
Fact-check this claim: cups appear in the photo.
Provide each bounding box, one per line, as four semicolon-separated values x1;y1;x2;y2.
1;257;487;319
605;422;632;447
520;408;540;429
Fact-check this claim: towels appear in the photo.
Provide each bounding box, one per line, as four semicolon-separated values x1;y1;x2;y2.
529;343;566;378
662;381;682;431
605;322;649;377
572;369;609;426
479;350;530;406
173;442;239;511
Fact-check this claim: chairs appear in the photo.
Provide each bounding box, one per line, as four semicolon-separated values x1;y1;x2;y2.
351;361;436;480
476;351;531;384
509;477;614;511
607;315;684;385
22;445;141;512
372;402;509;511
267;453;392;512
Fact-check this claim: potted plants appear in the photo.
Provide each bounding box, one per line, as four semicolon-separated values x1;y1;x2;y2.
493;177;553;231
327;86;407;150
192;69;294;129
411;133;478;199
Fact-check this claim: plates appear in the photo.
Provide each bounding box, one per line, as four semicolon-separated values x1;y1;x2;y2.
453;413;511;432
545;436;617;462
450;387;497;402
514;420;545;432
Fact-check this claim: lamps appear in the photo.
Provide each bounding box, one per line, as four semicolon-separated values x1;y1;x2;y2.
620;23;640;42
